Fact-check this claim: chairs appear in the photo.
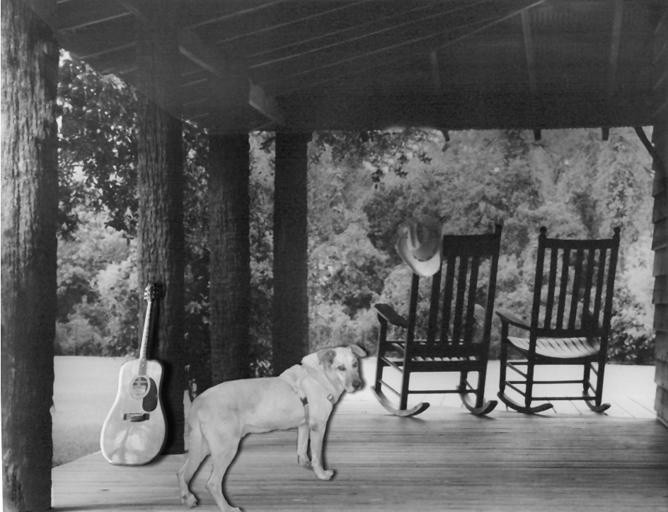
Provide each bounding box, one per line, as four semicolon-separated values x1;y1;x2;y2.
371;224;502;416
496;226;621;415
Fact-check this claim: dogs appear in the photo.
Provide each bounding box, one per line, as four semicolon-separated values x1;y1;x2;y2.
176;342;366;512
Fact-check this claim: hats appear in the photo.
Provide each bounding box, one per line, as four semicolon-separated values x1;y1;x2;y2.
395;215;442;278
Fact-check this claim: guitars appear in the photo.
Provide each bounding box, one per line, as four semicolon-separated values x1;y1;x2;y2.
101;282;167;465
